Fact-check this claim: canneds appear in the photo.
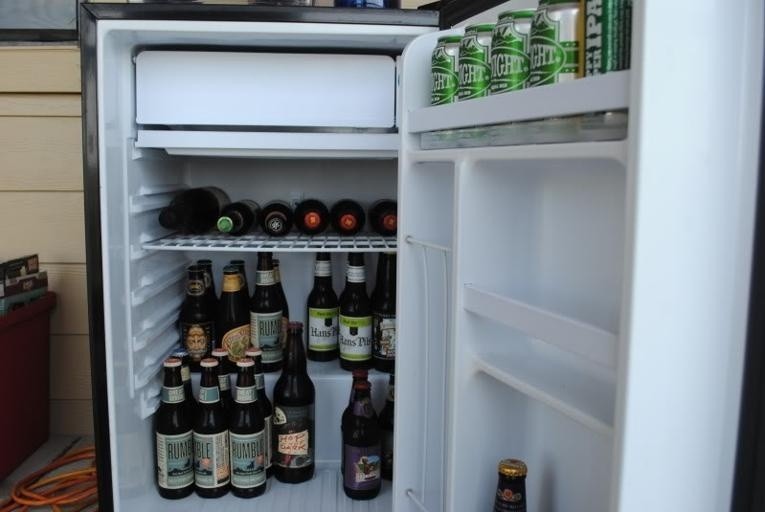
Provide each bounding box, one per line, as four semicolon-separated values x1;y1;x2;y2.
429;1;633;150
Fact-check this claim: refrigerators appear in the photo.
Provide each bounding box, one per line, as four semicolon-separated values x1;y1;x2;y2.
71;0;765;510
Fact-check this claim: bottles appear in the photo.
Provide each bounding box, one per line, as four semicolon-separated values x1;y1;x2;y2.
157;347;271;501
340;366;394;501
159;255;397;372
484;457;530;511
157;186;399;240
271;321;316;484
